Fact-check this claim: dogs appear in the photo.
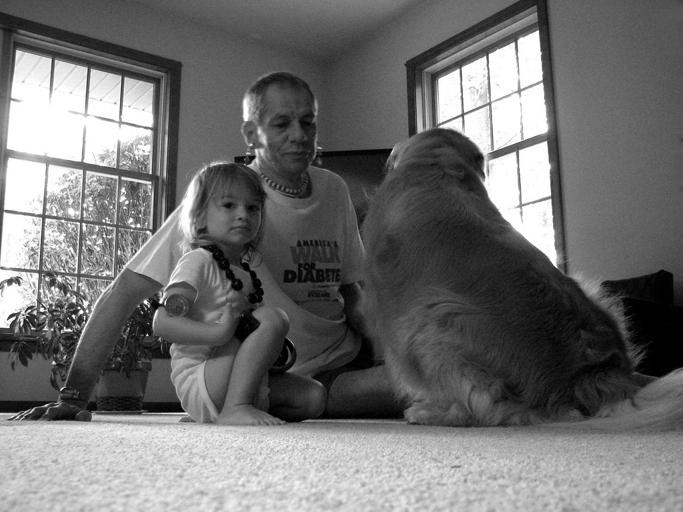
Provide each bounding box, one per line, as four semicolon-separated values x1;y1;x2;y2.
355;128;647;427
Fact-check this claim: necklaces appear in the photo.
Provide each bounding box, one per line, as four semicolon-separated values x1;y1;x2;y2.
256;168;310;195
191;239;265;305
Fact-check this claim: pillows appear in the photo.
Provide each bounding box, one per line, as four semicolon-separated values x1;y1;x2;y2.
600;269;682;376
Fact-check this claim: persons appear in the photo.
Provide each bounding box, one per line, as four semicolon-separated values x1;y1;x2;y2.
7;71;409;422
152;162;328;426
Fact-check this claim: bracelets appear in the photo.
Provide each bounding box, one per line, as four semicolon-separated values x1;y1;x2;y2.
59;387;89;403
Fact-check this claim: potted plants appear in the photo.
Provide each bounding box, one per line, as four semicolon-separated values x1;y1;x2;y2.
0;271;168;414
